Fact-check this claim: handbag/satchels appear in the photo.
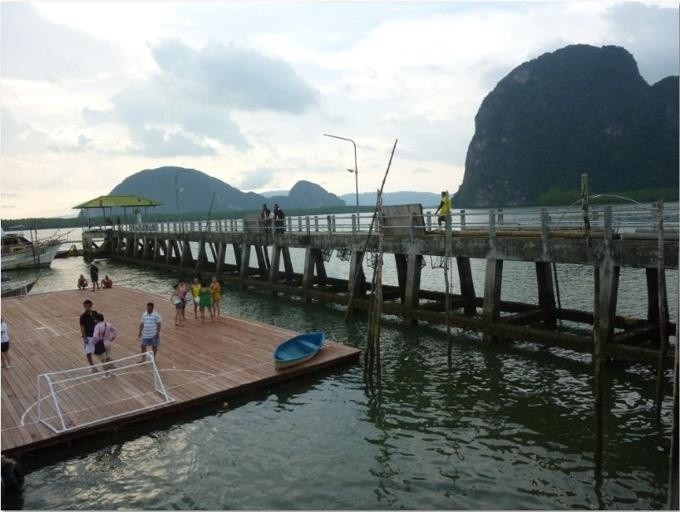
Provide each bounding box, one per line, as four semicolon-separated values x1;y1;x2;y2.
95;339;106;355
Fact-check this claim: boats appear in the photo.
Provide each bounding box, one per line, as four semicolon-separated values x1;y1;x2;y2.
1;273;43;296
274;331;325;371
1;226;65;271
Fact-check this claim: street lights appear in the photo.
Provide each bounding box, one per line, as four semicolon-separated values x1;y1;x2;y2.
175;186;186;233
348;168;361;231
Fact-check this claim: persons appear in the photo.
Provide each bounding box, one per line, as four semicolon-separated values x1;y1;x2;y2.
138;301;163;364
173;275;223;326
87;313;120;380
77;274;89;290
78;299;99;371
1;316;12;368
259;201;272;233
434;189;453;231
100;275;113;289
89;264;100;292
272;203;286;233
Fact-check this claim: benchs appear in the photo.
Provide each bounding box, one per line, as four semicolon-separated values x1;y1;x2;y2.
298;336;317;356
277;350;295;364
